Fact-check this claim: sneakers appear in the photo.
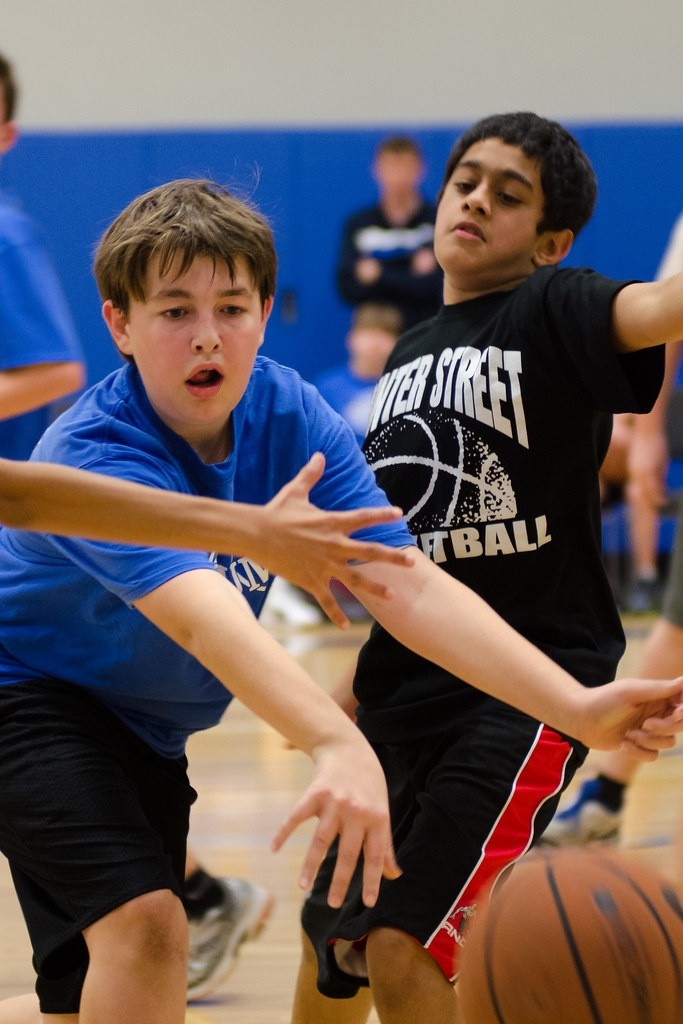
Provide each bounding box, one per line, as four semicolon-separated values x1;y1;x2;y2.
184;874;277;1005
537;777;625;846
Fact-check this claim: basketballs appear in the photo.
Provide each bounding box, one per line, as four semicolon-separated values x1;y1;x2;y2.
451;847;683;1024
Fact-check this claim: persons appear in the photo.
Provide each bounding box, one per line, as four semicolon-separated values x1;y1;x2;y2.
292;112;683;1024
339;141;443;380
0;57;276;1002
0;178;683;1023
599;414;668;614
0;452;413;629
544;222;683;851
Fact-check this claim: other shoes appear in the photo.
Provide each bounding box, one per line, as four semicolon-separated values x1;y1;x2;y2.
617;577;664;619
